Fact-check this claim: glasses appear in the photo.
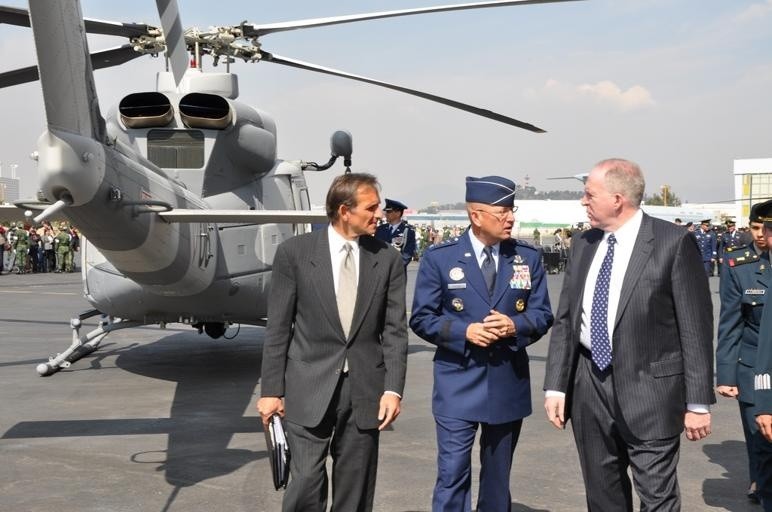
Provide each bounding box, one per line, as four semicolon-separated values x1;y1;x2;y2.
473;205;519;222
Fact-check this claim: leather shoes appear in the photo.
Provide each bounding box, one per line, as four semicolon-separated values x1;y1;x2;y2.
747;490;761;504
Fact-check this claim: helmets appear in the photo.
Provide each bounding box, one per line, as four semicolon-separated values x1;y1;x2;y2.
60;223;74;230
9;220;25;229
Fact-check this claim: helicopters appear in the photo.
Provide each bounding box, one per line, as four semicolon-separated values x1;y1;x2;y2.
7;0;580;375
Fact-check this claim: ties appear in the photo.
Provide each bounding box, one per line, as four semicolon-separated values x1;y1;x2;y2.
389;226;393;234
481;246;497;301
590;235;615;371
337;243;358;374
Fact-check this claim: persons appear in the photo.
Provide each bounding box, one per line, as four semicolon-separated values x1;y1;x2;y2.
0;220;78;275
374;197;415;283
673;216;749;278
542;158;718;512
717;202;766;502
412;224;466;262
409;175;554;512
255;172;408;511
750;264;772;442
714;199;771;512
553;223;584;272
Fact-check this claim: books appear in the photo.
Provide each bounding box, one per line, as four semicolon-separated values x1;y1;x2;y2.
262;412;291;491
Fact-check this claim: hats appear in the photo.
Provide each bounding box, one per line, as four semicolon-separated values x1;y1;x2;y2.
382;198;408;212
750;200;772;229
725;220;736;226
701;219;711;225
465;176;516;206
